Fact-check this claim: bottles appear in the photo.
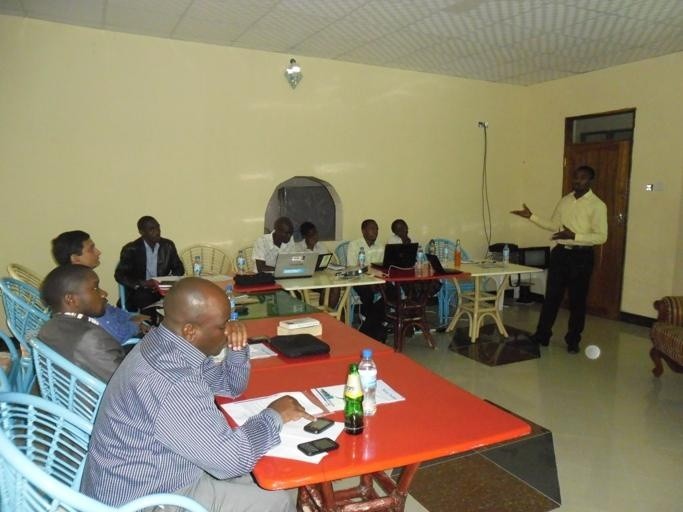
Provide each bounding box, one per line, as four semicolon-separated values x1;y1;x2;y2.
193;257;202;278
346;348;378;435
417;237;461;269
358;247;366;268
225;286;239;321
236;250;246;276
502;244;509;266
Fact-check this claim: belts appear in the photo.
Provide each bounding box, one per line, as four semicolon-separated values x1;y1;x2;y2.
557;243;592;250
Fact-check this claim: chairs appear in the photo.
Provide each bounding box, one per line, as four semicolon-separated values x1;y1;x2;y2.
649;292;683;376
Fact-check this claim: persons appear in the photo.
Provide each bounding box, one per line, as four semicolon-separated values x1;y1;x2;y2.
80;276;317;512
113;215;184;326
346;219;384;336
509;164;608;353
289;221;339;308
51;229;152;346
386;218;442;334
36;263;126;386
250;216;304;304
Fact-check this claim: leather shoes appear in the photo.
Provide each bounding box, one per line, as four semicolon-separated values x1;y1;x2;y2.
568;342;580;354
527;336;549;346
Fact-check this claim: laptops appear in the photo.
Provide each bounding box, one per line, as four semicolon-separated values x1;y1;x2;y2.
370;243;419;268
426;252;462;275
261;251;320;278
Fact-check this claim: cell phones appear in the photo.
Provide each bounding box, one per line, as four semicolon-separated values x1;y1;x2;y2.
297;437;338;455
247;334;270;344
304;417;335;434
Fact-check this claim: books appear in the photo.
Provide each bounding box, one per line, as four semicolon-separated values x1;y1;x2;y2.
276;325;322;337
309;379;405;414
278;317;319;329
157;280;175;289
214;342;277;364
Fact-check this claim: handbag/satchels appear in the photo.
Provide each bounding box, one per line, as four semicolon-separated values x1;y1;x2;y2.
234;272;274;287
